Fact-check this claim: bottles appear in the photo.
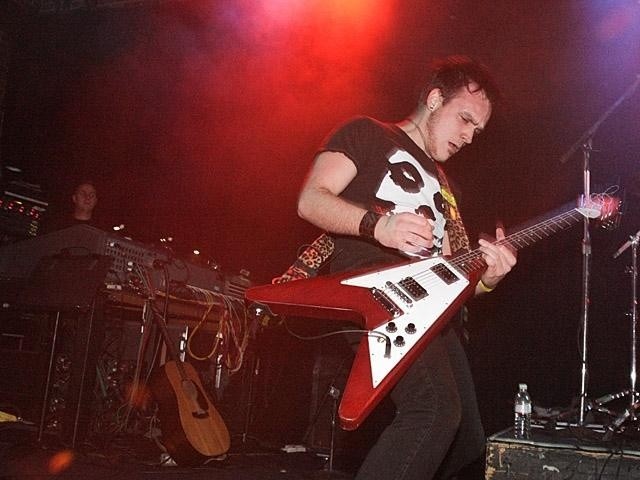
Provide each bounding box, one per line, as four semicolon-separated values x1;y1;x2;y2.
513;384;532;441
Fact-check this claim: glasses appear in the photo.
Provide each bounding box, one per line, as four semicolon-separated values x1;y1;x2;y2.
77;191;97;200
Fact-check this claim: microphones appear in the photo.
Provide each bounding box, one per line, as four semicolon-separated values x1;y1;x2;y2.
613;231;640;259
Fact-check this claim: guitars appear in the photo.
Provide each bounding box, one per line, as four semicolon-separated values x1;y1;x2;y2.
126;257;231;470
243;190;624;432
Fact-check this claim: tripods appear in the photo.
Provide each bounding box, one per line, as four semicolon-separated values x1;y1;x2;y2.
553;246;639;441
529;76;630;441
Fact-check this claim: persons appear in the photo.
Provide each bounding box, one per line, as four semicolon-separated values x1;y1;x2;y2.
37;179;99;235
295;52;519;480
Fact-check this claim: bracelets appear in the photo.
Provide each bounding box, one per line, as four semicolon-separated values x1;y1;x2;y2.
357;209;384;238
479;275;496;294
